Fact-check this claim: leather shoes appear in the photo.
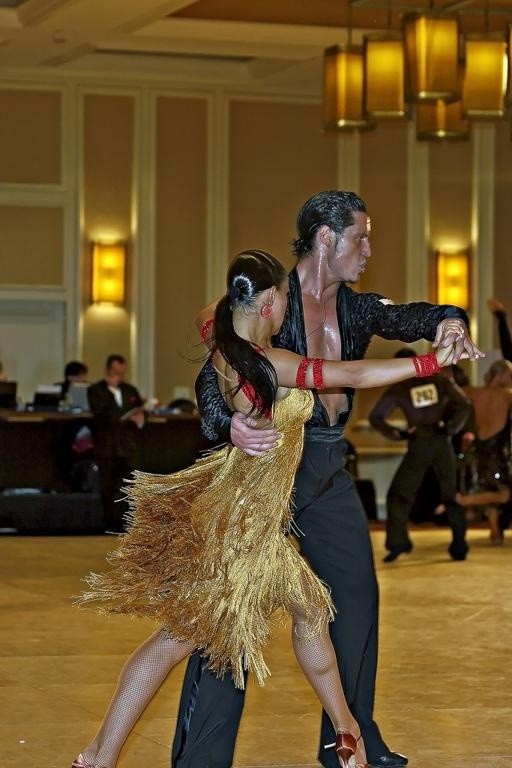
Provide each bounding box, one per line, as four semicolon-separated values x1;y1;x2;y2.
384;541;413;562
448;544;468;560
370;747;407;766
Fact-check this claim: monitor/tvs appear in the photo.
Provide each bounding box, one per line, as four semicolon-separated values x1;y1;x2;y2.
68;381;90;410
0;381;17;406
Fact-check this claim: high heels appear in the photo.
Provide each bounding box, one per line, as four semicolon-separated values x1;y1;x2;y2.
332;726;368;766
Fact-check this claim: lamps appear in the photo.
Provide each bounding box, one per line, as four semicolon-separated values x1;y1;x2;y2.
84;230;133;316
313;0;512;148
423;236;476;319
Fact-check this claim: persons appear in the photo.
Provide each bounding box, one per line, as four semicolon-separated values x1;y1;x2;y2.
0;363;24;411
69;249;479;767
86;354;146;536
368;348;473;562
487;297;512;539
434;357;512;545
53;361;87;407
172;190;487;767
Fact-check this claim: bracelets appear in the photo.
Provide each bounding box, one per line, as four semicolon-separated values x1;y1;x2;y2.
410;352;440;378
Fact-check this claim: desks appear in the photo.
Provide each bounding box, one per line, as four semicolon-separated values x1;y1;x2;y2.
343;443;415;531
0;398;209;539
